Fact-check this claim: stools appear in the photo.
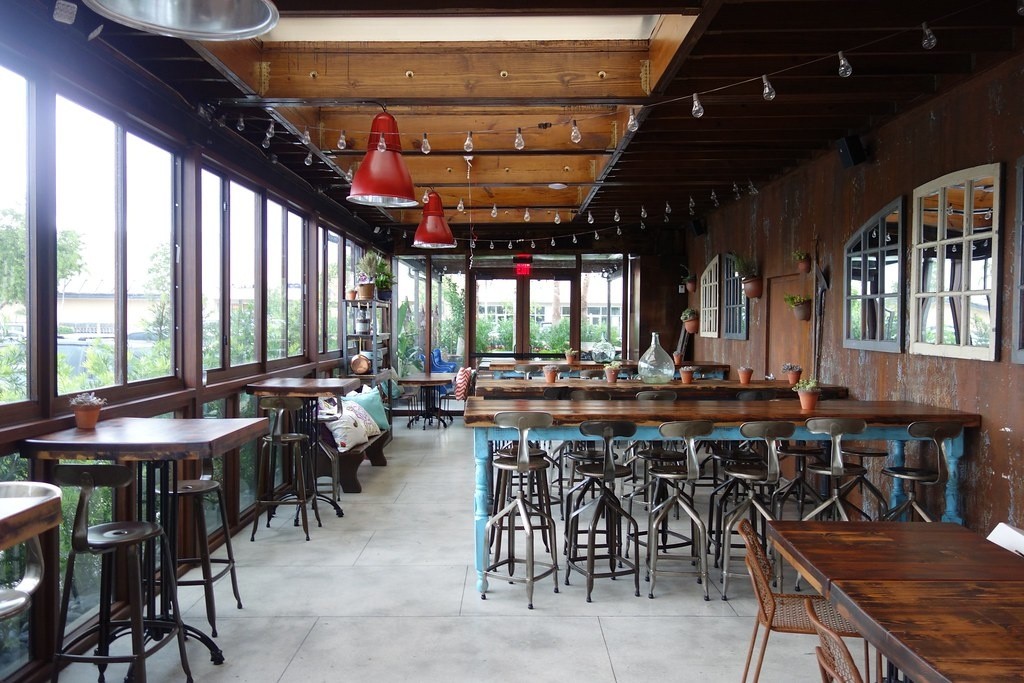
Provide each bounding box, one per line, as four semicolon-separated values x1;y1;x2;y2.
378;366;465;430
52;397;343;683
481;386;963;609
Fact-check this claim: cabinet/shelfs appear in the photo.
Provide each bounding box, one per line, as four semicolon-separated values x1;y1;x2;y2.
342;299;392;388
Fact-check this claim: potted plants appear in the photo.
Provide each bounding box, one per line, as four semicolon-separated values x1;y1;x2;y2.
69;391;108;429
346;282;357;300
355;307;371;335
543;248;821;410
355;249;398;303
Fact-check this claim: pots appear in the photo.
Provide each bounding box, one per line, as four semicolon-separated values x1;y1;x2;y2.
351;337;372;374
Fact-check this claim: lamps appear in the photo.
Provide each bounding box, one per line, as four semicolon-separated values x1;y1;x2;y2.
343;107;532;277
83;0;280;42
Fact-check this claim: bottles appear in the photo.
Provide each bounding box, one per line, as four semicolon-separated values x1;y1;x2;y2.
638;333;675;384
591;331;615;363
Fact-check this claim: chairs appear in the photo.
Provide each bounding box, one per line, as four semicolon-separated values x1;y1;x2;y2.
0;481;63;683
510;364;634;382
737;518;882;683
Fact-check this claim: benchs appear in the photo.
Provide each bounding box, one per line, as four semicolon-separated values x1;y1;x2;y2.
420;349;456;393
295;404;389;492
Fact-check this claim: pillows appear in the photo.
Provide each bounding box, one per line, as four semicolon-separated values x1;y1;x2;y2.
469;369;477;392
453;366;471;400
311;368;406;453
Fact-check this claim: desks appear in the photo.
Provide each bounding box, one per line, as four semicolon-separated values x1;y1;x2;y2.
766;519;1024;683
19;415;269;667
247;376;360;526
478;355;730;372
397;374;456;430
475;378;848;398
463;396;981;592
